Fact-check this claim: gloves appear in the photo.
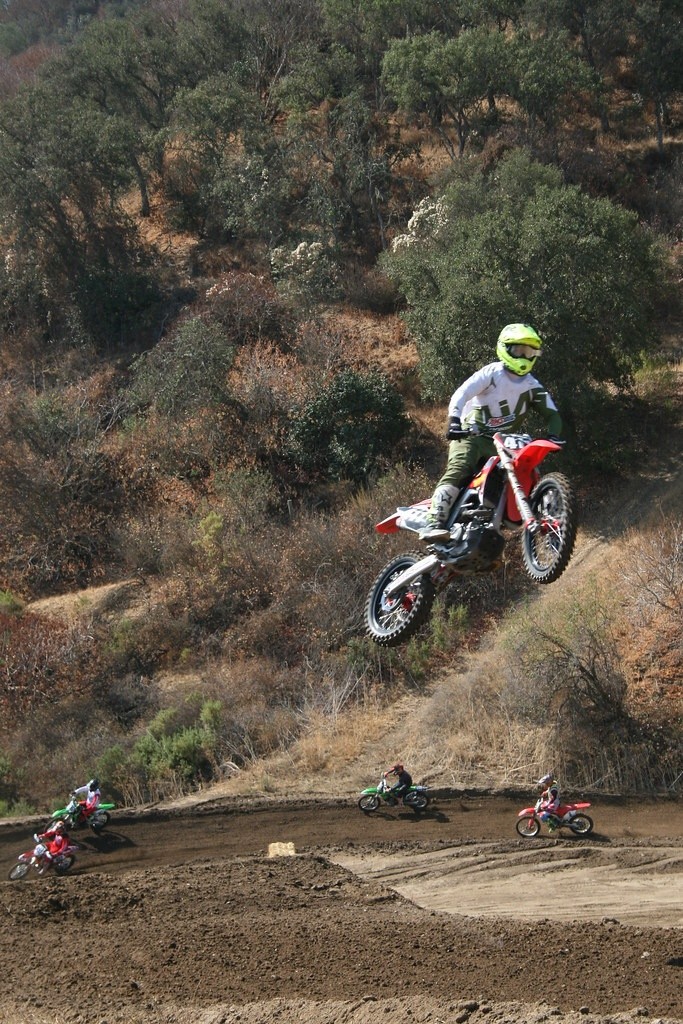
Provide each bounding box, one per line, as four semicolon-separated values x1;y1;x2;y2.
548;434;560;454
446;416;463;440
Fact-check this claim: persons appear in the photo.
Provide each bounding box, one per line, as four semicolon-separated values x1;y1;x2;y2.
419;324;562;541
71;778;101;832
38;821;69;876
538;775;560;833
384;763;412;806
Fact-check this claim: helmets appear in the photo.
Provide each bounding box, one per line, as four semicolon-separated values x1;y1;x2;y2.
87;779;99;791
56;821;64;828
393;765;403;771
539;775;553;783
496;323;542;376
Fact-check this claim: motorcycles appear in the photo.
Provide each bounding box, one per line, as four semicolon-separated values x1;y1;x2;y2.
358;774;430;813
362;426;579;648
46;794;115;836
515;795;594;838
8;834;79;881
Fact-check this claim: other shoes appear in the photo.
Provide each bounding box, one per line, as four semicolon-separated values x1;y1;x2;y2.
418;514;450;543
394;802;404;809
549;828;554;833
38;869;44;876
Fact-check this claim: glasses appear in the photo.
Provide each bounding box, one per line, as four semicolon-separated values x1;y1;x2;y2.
507;344;541;359
